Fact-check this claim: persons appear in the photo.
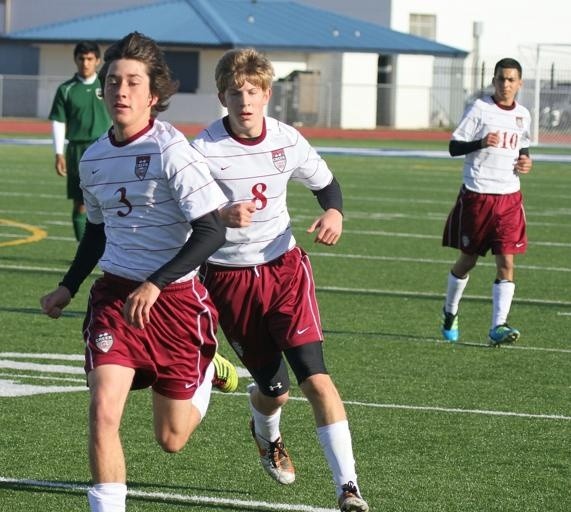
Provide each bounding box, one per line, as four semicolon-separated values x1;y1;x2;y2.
48;41;112;241
41;30;239;512
186;46;371;511
441;58;532;346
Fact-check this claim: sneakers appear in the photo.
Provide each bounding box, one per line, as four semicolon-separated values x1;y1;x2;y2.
211;351;239;394
486;323;521;348
336;485;369;512
249;415;297;485
440;303;459;342
